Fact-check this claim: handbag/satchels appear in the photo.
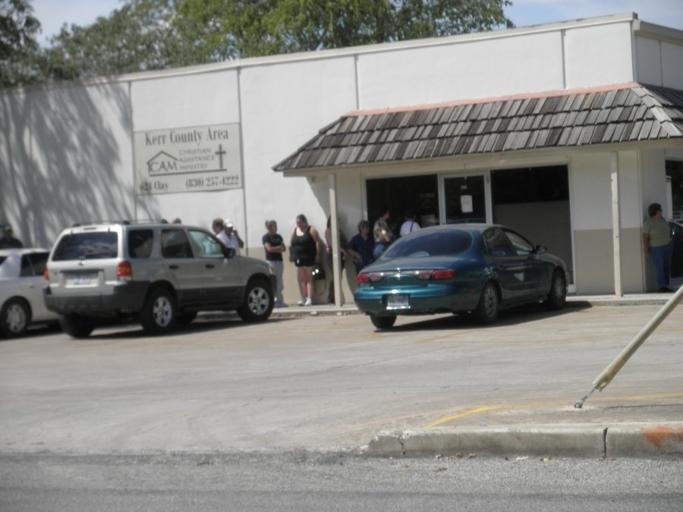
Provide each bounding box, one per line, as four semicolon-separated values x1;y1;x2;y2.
312;264;325;280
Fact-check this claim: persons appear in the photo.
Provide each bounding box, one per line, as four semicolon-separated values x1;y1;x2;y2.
641;201;674;294
347;210;423;275
290;214;320;306
666;204;683;282
211;218;245;257
262;220;288;307
0;224;25;250
324;216;349;306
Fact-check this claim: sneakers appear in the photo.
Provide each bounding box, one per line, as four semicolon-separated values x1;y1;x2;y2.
298;297;312;306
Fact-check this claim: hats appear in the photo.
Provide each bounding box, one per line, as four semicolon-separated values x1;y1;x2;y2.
223;220;233;228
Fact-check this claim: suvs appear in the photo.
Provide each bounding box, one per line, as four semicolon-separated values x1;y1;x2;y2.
43;221;278;338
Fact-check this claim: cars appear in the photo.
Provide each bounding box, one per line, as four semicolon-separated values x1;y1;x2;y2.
353;223;569;331
0;248;59;337
